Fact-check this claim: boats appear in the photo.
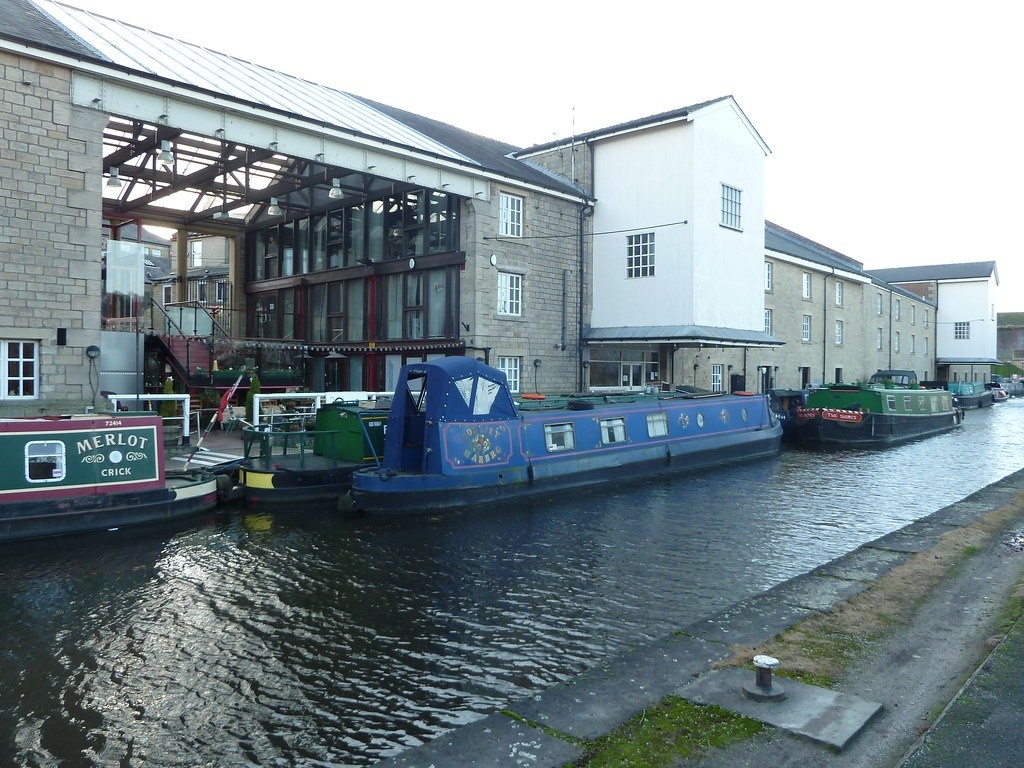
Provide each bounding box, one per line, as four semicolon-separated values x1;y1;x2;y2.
0;416;217;540
922;376;1023;410
229;356;785;518
767;371;962;448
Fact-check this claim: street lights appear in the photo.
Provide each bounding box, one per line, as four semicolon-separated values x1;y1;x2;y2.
295;351;315;391
324;349;346;391
762;366;767;392
